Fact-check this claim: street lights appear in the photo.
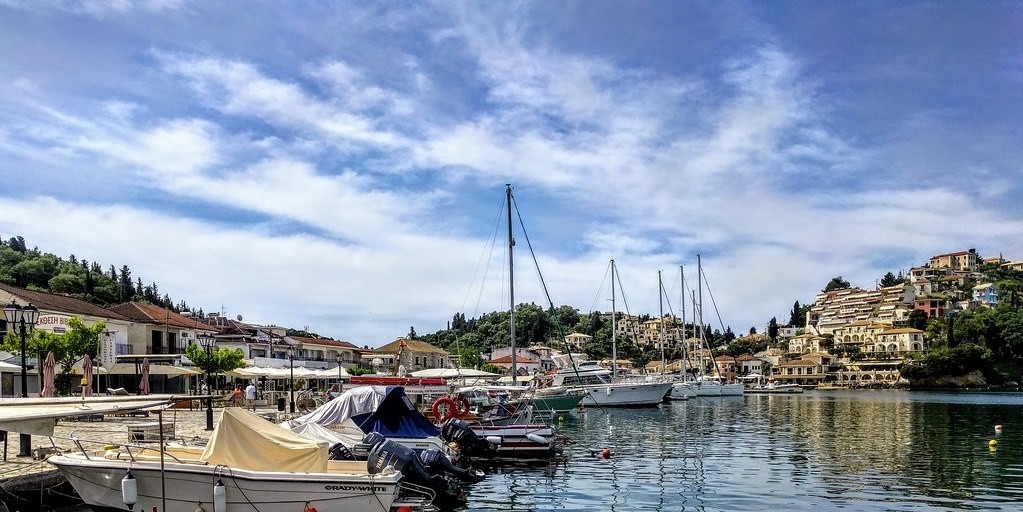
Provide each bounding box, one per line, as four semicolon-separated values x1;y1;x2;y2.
198;331;218;432
286;344;296;413
336;353;344;393
3;297;41;456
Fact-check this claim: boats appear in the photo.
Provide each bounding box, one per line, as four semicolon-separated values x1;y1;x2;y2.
274;384;486;506
348;374;571;464
44;405;438;512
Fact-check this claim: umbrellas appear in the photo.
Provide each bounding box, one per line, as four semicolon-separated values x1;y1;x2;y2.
82;354;93;397
139;358;149;394
40;351;55;397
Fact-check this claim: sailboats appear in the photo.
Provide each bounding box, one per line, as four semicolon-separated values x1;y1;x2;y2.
552;258;673;408
655;254;746;400
459;184;590;417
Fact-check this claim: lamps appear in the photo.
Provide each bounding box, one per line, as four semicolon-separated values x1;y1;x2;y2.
989;440;997;453
81;378;87;386
995;424;1002;435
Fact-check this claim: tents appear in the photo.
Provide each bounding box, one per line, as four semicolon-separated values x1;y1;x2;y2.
245;366;354;391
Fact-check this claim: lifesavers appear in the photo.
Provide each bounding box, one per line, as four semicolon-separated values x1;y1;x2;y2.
451;396;470;417
432;398;456;422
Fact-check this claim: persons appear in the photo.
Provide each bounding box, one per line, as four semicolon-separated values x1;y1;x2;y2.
246;382;256;412
234;383;242;407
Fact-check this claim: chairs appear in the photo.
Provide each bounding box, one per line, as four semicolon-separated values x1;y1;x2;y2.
61;388;148;422
